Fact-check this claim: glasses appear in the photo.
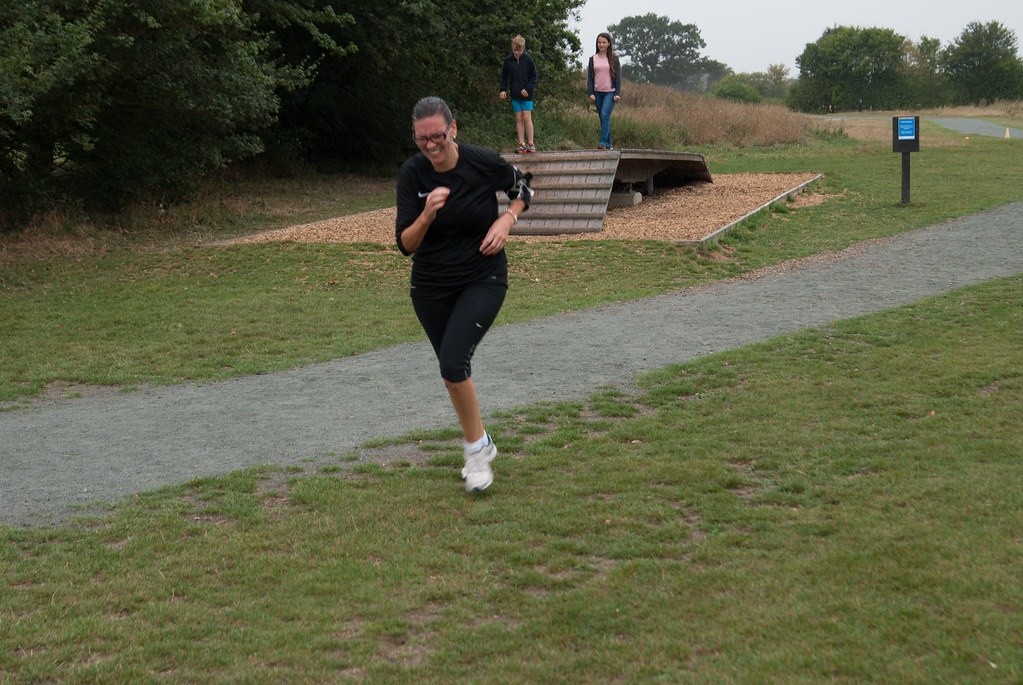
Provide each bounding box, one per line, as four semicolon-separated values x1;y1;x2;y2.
410;121;452;145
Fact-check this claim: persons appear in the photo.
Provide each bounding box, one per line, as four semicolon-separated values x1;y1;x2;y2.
587;33;621;149
395;96;534;490
499;34;537;154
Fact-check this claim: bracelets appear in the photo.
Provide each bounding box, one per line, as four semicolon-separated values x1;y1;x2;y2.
503;208;521;224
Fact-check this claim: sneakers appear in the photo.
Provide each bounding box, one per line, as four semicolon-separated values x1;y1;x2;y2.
526;143;535;154
515;143;527;155
461;434;497;493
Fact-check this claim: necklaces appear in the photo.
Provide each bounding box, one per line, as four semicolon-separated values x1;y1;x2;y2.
431;159;438;172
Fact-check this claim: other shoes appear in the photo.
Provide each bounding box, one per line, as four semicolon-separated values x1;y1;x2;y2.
598;143;614;150
